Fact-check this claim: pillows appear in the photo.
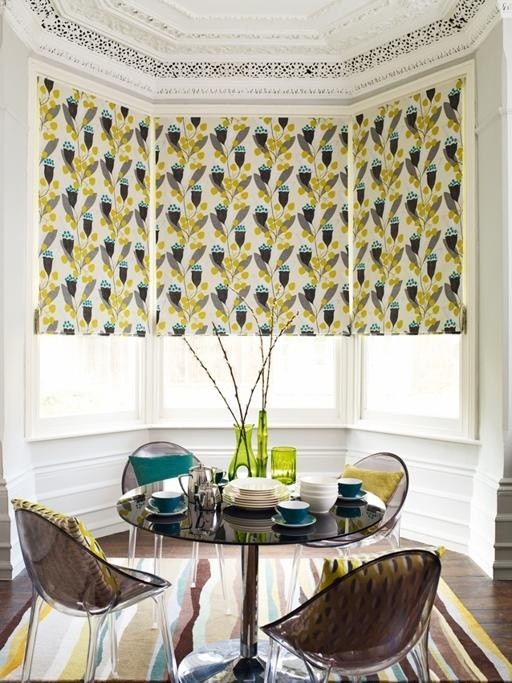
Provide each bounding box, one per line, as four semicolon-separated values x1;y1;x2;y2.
290;546;444;652
330;462;403;531
130;457;191;486
12;498;119;609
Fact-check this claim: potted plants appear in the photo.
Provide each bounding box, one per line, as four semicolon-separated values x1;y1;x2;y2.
183;287;299;478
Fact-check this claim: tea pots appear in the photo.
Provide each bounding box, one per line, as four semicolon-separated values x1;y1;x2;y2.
178;462;215;504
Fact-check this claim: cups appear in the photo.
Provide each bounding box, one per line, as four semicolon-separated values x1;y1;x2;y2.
148;523;181;537
215;471;226;484
337;478;362;497
271;446;296;485
275;501;310;524
336;506;362;518
148;491;182;513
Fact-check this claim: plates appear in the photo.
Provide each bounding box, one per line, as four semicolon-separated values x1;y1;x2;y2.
222;477;291;508
271;513;316;528
145;504;188;516
337;490;367;500
221;507;277;531
273;529;317;537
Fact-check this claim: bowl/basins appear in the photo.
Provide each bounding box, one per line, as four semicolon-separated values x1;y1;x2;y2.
307;514;338;540
300;476;339;513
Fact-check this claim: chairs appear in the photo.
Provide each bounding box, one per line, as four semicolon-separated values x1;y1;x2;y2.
12;506;181;682
288;451;410;611
260;550;441;682
119;442;231;627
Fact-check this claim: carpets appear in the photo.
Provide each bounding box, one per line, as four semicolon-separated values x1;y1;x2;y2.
1;554;511;682
